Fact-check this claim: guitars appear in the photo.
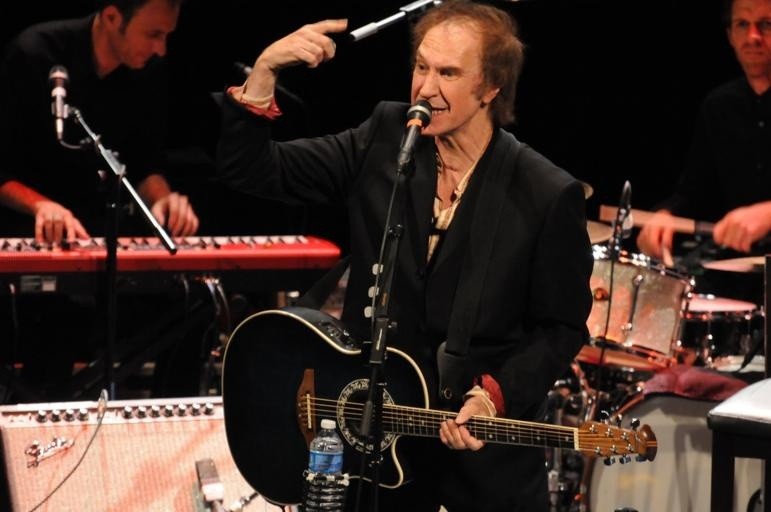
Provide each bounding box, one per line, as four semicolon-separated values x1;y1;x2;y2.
222;305;654;504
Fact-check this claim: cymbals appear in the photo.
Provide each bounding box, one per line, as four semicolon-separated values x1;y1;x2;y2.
702;255;767;273
586;218;616;245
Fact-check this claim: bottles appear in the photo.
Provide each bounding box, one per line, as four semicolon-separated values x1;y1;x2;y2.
304;419;344;511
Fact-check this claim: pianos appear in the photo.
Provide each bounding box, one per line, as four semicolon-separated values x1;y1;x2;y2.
1;226;342;275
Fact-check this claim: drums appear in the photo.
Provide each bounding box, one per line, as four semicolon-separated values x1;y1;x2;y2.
712;352;771;378
588;245;693;366
682;289;758;364
583;378;765;512
571;343;657;423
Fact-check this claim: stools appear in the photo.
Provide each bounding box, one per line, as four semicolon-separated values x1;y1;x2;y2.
706;375;771;512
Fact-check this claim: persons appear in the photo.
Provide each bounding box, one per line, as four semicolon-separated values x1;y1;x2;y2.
213;1;593;511
635;1;771;259
0;0;221;397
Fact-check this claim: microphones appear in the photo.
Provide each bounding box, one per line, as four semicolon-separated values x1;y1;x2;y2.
46;61;68;138
615;179;635;241
397;100;430;168
